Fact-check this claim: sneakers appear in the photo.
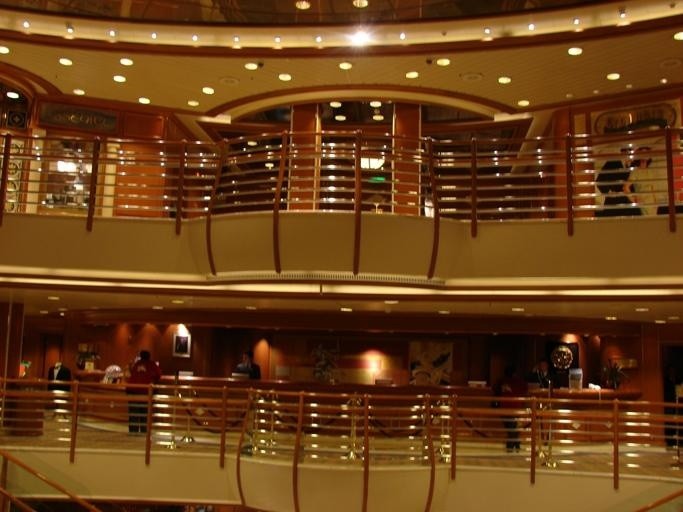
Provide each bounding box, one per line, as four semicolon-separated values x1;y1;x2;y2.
507;441;521;453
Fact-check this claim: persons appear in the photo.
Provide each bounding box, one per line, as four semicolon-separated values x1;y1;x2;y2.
491;359;529;453
234;349;261;378
528;359;558;387
621;144;660;215
594;147;638;215
43;361;71;409
124;350;163;433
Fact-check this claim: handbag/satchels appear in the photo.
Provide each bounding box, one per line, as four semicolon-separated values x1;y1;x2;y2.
490;383;501;408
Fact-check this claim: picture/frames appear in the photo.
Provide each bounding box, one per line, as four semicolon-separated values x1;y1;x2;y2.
170;331;191;358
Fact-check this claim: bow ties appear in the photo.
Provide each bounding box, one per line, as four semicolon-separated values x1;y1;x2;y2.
53;366;60;369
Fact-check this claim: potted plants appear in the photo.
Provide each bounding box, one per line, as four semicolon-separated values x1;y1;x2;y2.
601;357;628;390
